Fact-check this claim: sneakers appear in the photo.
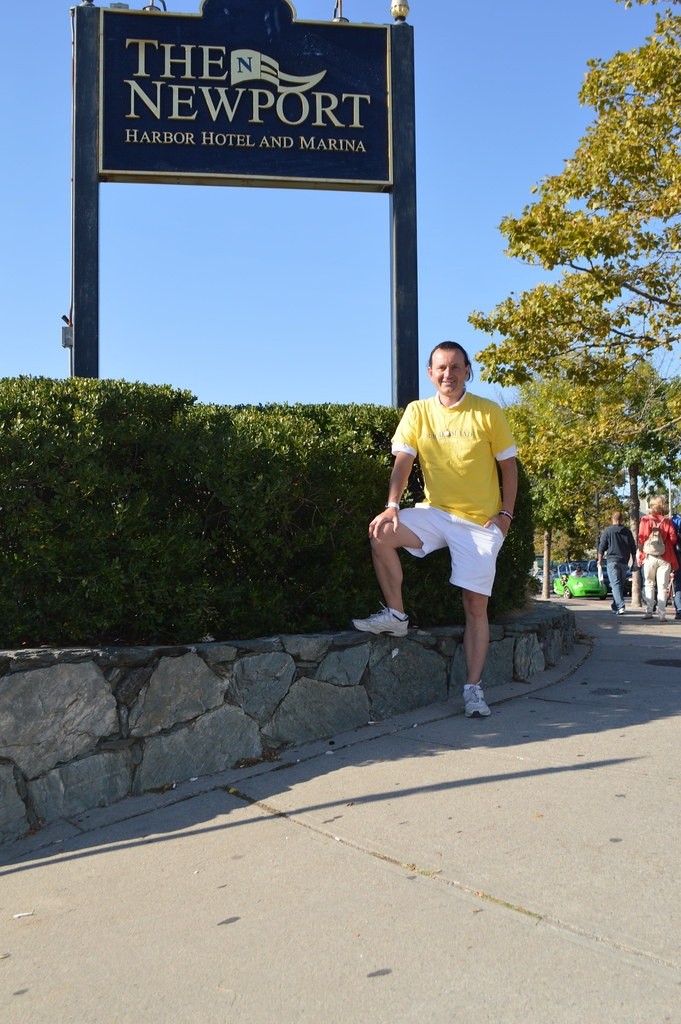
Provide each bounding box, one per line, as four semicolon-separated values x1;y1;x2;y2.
462;679;491;717
353;602;409;638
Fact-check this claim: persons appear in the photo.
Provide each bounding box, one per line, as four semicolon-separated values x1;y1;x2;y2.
569;565;586;577
598;510;636;616
639;492;681;621
350;342;521;721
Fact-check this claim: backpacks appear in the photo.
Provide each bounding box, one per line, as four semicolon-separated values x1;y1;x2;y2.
643;515;665;557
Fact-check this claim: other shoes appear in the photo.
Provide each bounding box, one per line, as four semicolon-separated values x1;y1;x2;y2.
611;604;617;614
642;613;652;619
616;607;626;614
659;615;667;622
675;610;681;619
653;600;657;611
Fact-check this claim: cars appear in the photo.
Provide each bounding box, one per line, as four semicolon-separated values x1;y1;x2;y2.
553;571;608;600
535;558;643;598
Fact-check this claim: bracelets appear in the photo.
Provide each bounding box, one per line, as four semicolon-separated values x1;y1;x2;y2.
499;509;513;519
385;502;400;511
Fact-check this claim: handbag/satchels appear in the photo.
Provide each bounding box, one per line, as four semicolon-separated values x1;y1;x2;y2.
597;564;604;587
